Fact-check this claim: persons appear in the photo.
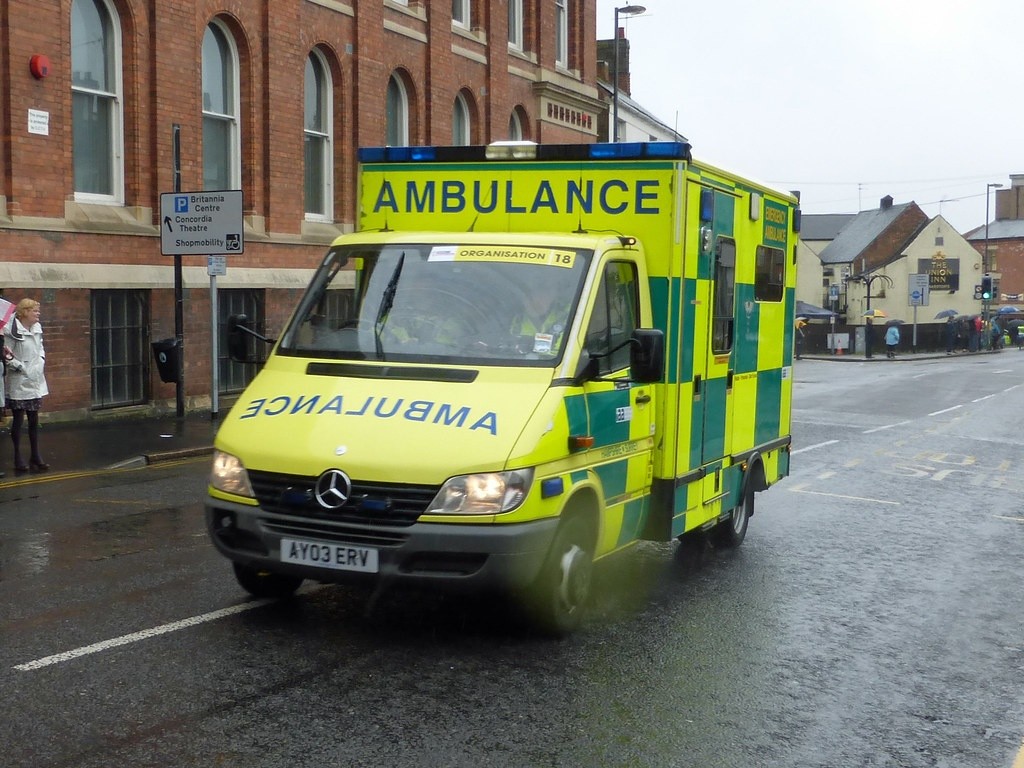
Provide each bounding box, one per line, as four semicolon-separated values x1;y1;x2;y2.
511;280;566;354
373;272;459;344
0;299;50;476
942;316;1019;355
793;323;805;361
884;327;899;358
864;318;873;357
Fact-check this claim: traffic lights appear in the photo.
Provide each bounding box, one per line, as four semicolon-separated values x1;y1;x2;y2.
982;277;992;300
993;285;997;298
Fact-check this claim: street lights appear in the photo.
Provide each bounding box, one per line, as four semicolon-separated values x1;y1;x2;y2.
613;5;646;142
983;184;1003;348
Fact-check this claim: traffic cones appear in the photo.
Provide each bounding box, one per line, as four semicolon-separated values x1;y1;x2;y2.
835;339;843;356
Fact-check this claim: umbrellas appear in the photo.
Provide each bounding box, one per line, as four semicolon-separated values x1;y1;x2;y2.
937;305;1024;327
883;319;907;326
796;318;810;322
861;309;885;317
795;300;840;317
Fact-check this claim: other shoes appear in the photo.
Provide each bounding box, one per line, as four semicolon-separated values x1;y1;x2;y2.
28;460;51;471
962;348;967;352
14;463;31;472
887;351;897;358
796;356;802;360
867;356;875;358
947;351;956;355
0;472;6;478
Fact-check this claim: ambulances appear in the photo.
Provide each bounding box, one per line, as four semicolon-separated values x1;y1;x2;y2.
204;140;802;639
